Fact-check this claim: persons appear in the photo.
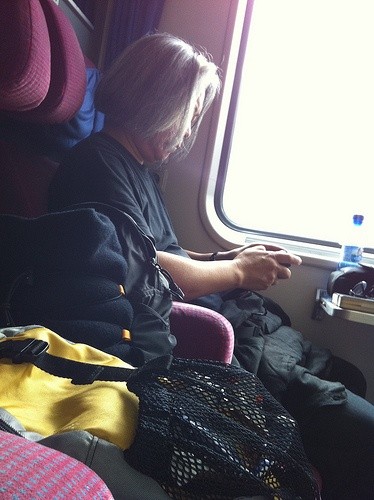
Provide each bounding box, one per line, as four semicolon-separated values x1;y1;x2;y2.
49;34;374;500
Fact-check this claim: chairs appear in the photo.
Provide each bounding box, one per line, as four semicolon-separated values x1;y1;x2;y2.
0;0;324;500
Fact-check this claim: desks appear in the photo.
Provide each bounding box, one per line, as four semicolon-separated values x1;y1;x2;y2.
312;288;374;326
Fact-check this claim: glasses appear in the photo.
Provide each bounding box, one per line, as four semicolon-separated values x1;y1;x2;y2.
350;281;374;300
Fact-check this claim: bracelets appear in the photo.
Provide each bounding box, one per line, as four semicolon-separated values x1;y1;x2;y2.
209;251;219;261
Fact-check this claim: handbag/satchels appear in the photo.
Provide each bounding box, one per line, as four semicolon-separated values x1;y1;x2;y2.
328;266;374;297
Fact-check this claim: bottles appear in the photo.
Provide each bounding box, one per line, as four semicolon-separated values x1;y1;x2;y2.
341;214;364;263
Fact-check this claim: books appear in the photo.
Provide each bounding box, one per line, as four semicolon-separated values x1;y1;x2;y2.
331;291;374;314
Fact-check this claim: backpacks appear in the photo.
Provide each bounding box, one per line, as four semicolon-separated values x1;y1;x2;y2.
0;200;185;364
0;325;320;500
